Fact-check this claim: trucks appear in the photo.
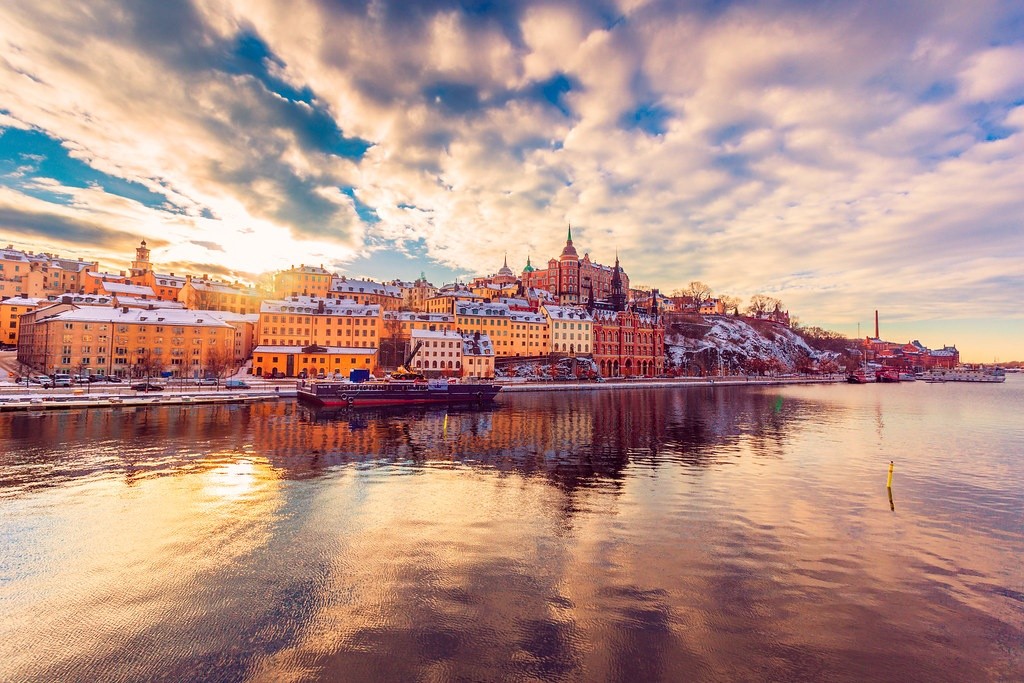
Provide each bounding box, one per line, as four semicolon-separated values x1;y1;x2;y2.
226;380;251;390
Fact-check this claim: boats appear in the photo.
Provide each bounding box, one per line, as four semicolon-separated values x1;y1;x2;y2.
915;364;1006;383
846;367;916;383
296;379;503;409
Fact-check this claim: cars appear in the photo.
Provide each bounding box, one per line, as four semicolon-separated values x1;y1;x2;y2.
635;374;674;378
263;372;285;379
317;373;325;379
595;378;606;383
196;378;220;385
137;383;162;391
106;375;121;382
15;377;31;383
90;374;107;382
30;373;74;387
71;374;90;384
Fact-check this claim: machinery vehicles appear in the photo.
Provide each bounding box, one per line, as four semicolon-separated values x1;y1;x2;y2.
392;342;425;380
